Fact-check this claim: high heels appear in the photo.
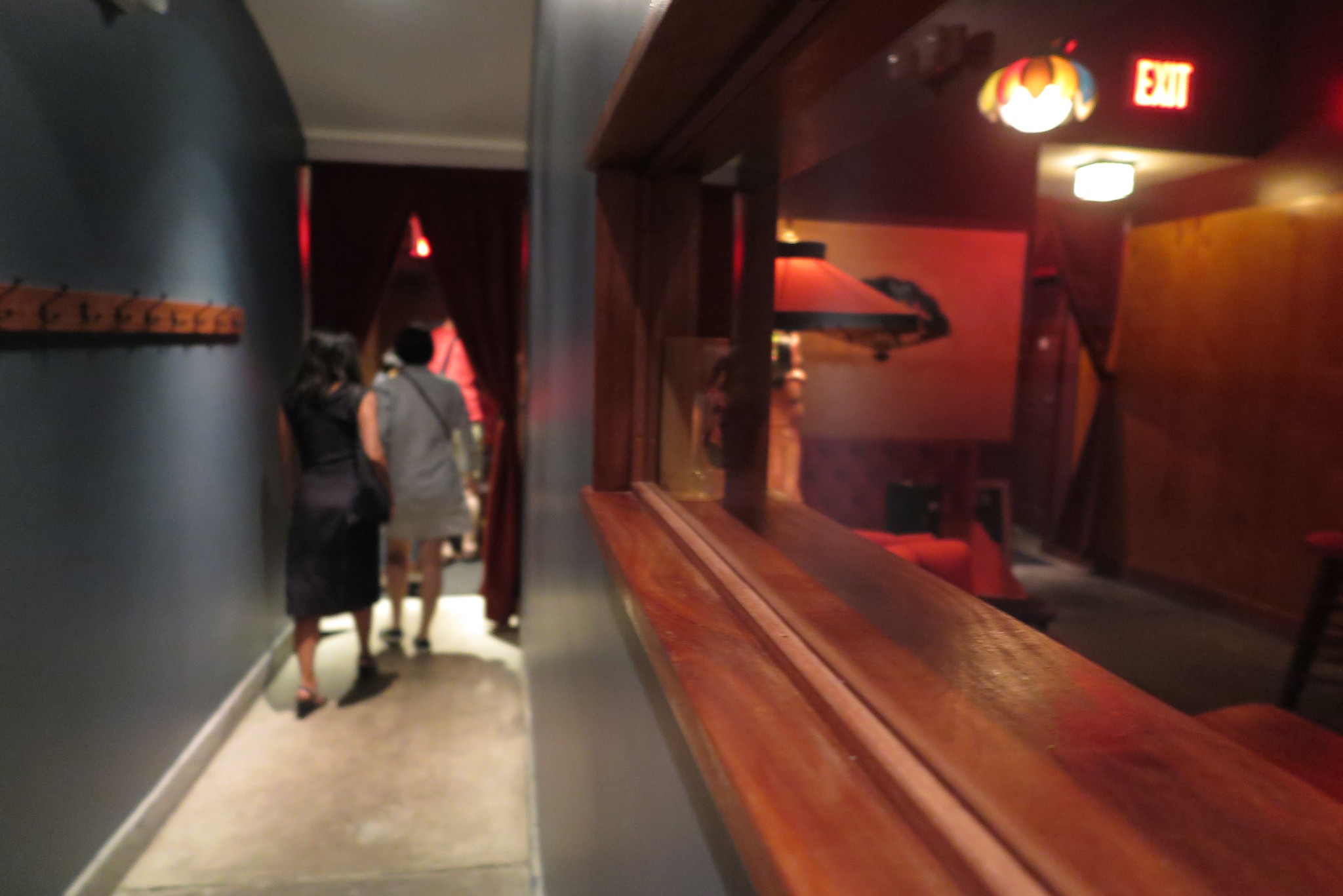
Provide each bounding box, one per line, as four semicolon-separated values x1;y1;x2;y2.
297;688;322;718
357;655;377;683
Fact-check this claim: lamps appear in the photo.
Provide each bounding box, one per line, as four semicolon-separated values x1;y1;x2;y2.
977;32;1098;133
771;226;916;337
1074;162;1136;203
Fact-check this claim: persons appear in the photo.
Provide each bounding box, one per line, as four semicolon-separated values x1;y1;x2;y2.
279;329;396;717
430;316;484;567
375;327;467;647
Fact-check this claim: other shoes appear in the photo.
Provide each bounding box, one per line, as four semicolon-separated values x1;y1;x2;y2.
414;637;430;650
379;628;402;646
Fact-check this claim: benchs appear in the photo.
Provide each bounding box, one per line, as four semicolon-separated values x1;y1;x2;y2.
1193;699;1343;803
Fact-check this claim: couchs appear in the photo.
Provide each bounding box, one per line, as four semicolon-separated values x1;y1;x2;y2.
856;529;1058;633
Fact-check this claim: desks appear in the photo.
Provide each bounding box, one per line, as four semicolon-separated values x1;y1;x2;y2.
1278;529;1343;724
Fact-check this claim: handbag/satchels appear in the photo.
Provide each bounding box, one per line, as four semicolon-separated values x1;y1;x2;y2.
354;456;391;524
